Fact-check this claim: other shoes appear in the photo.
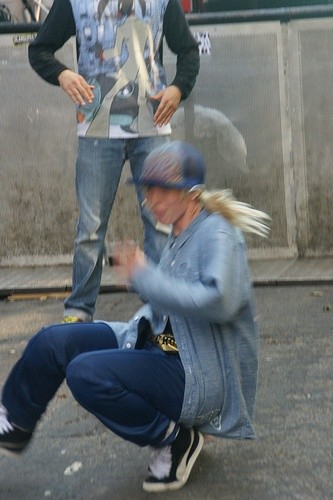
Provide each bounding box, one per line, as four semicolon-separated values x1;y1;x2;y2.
60;316;82;322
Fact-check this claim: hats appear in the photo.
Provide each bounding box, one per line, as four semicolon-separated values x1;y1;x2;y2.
126;142;204;189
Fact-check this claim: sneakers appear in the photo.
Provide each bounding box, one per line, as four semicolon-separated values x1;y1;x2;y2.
142;423;204;492
0;401;33;450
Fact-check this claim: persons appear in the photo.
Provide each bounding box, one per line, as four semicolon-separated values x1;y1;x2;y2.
0;142;269;490
28;0;200;323
